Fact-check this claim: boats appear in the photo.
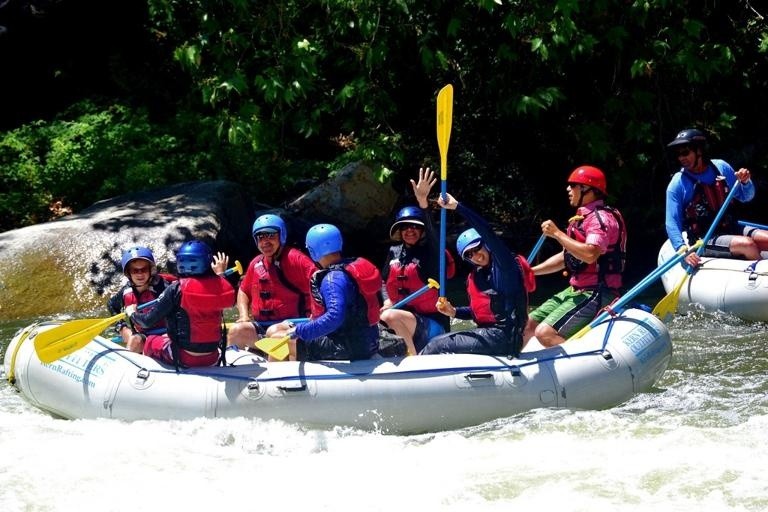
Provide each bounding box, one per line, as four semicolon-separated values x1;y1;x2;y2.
657;235;768;324
3;295;674;437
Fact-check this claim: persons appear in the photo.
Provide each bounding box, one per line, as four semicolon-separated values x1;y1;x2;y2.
126;240;236;368
417;193;537;357
522;165;627;350
379;167;456;357
666;127;768;268
226;215;320;350
106;245;179;353
266;224;382;363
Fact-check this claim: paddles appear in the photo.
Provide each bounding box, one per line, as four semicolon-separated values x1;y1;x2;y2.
255;321;310;361
652;179;742;321
437;84;454;309
35;260;244;363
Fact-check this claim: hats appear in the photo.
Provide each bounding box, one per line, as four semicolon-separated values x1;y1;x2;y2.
387;217;424;242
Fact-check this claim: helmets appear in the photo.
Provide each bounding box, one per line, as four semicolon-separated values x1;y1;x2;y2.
566;165;607;197
455;228;481;261
303;223;343;264
666;125;707;148
251;214;289;246
121;245;155;274
175;238;213;276
395;206;424;220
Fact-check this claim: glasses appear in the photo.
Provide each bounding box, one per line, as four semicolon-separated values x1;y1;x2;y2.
256;232;277;240
400;223;422;231
128;267;149;274
569;181;577;187
674;149;690;159
464;247;482;259
